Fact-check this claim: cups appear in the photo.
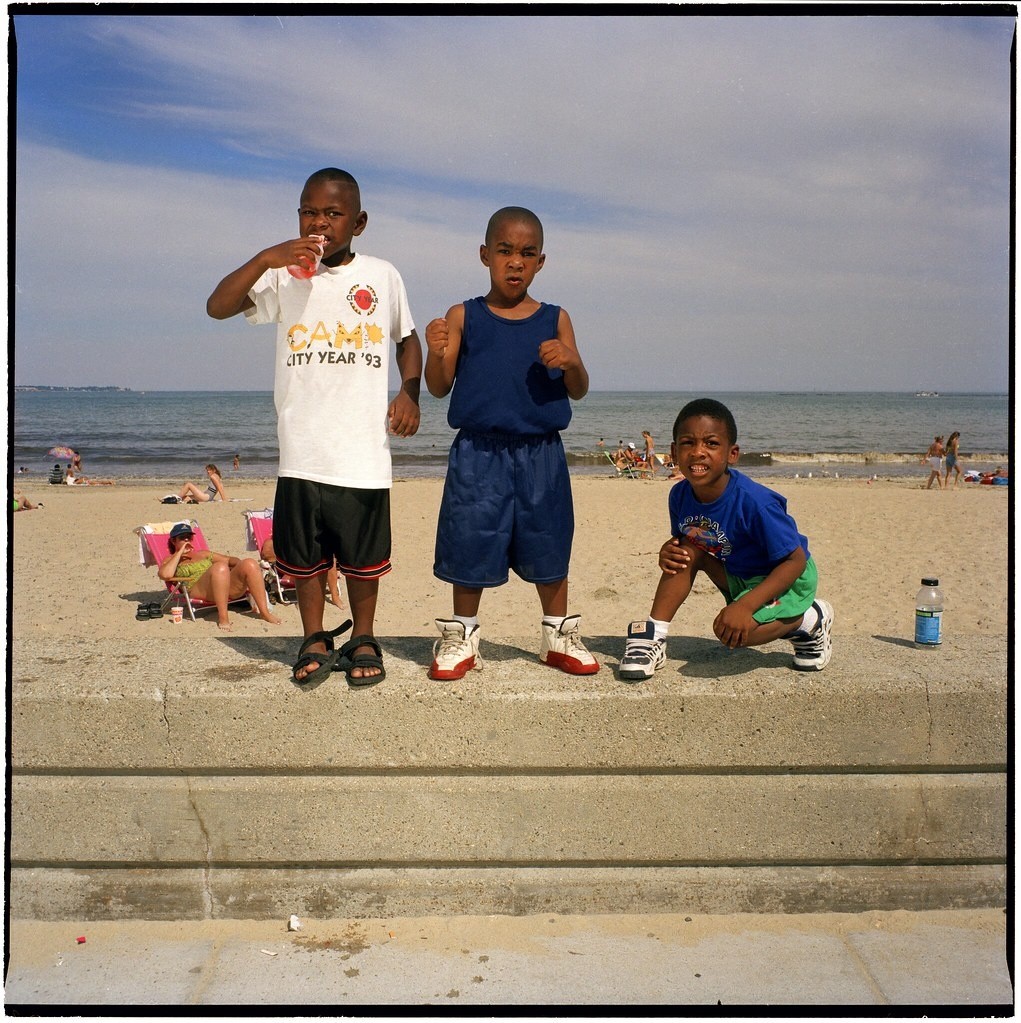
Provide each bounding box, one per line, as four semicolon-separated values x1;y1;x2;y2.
172;606;182;624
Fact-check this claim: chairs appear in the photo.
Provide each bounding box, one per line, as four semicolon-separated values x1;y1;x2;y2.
243;508;341;599
604;450;655;482
130;519;270;621
656;453;680;476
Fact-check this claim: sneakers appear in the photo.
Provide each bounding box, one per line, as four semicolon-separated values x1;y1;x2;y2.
618;620;667;679
539;615;600;675
430;615;484;680
780;599;834;672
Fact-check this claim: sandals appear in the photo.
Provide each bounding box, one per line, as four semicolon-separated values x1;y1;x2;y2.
135;601;149;621
150;602;163;618
339;635;385;685
292;619;352;684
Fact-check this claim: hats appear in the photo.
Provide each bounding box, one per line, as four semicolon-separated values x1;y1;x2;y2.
169;523;196;539
629;443;635;448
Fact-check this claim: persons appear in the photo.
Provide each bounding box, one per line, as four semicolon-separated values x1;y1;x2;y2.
978;465;1008;485
14;490;39;512
260;533;348;609
424;207;600;681
432;445;434;447
156;523;283;631
920;435;947;489
234;454;240;470
618;440;623;448
618;397;836;683
49;452;116;484
641;431;655;478
610;442;649;478
945;431;962;489
18;467;29;473
664;454;688;480
596;438;605;447
156;464;228;503
206;167;425;686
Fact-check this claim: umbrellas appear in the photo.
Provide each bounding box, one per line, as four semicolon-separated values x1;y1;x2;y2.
49;445;76;459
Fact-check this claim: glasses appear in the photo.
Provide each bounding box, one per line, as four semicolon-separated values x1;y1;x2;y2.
177;534;191;541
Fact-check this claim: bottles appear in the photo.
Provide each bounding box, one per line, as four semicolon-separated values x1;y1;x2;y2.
288;235;327;278
915;577;944;650
